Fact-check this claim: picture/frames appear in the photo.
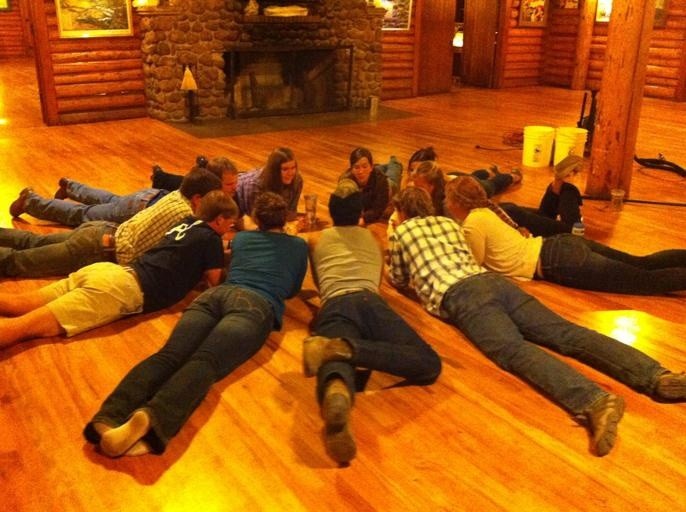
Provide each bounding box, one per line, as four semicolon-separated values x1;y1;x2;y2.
517;1;549;29
53;0;135;39
366;0;413;31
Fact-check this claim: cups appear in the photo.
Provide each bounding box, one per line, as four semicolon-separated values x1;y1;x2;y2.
611;189;625;211
303;192;318;225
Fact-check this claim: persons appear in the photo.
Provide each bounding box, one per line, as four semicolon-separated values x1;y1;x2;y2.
150;145;304;221
0;166;224;277
0;190;240;347
8;155;239;230
379;185;686;457
337;146;403;226
400;145;523;203
443;174;686;292
410;154;583;235
301;184;442;463
78;190;309;459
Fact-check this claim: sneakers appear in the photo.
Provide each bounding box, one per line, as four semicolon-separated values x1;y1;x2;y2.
511;167;523;179
655;372;686;399
489;164;500;175
581;392;625;456
555;154;581;179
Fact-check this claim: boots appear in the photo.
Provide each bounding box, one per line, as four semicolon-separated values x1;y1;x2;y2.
303;336;352;376
321;378;357;463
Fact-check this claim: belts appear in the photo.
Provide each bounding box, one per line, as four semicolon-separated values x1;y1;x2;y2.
110;223;118;262
140;190;159;211
120;262;140;287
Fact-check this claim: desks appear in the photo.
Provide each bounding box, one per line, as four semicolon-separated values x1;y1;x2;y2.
223;46;354;120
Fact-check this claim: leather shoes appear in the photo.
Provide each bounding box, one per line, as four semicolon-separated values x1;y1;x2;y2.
53;177;69;200
9;187;33;218
